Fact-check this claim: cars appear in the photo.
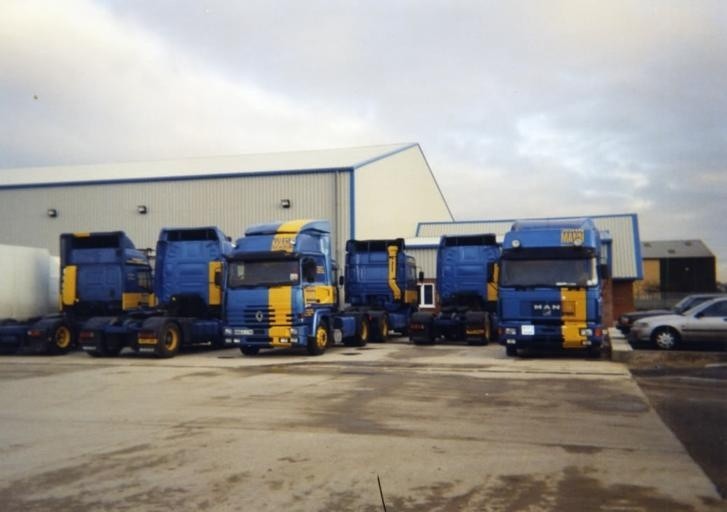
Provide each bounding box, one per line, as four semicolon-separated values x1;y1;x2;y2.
615;292;727;351
